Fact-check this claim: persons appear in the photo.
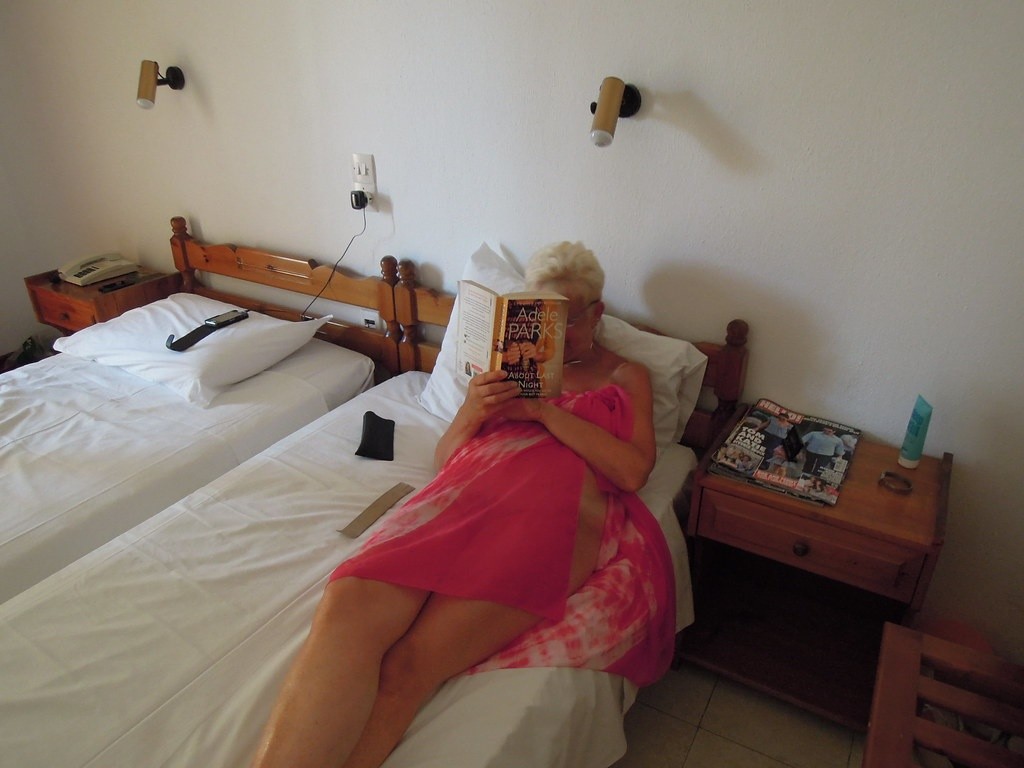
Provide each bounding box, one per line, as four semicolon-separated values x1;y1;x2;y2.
753;414;794;470
767;441;788;477
798;426;843;480
502;300;555;397
838;431;859;460
807;480;824;492
252;241;674;768
735;448;750;470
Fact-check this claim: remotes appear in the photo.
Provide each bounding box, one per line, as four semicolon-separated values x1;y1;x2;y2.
99;279;135;292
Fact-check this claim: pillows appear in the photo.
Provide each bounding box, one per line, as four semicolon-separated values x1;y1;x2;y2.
53;292;333;407
413;241;707;497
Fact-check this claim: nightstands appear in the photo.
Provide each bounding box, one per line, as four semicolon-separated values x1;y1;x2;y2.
673;401;954;732
24;264;184;337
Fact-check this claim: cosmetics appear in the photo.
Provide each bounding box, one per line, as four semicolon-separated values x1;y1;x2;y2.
896;394;933;470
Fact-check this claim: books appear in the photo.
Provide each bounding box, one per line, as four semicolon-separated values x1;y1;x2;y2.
707;397;862;507
453;280;569;399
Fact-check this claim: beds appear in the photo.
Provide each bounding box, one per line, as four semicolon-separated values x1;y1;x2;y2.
0;215;397;603
0;260;750;767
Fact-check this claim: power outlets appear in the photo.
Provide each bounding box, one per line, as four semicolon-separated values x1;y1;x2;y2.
353;182;379;212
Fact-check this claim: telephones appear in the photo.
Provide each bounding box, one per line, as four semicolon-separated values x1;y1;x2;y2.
58;251;140;287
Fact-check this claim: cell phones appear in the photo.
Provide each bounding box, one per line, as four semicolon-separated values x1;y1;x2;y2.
205;310;248;327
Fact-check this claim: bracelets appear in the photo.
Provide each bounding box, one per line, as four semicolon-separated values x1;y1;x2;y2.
878;470;913;494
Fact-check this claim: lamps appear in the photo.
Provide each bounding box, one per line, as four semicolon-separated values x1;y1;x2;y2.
137;59;185;109
589;76;641;147
